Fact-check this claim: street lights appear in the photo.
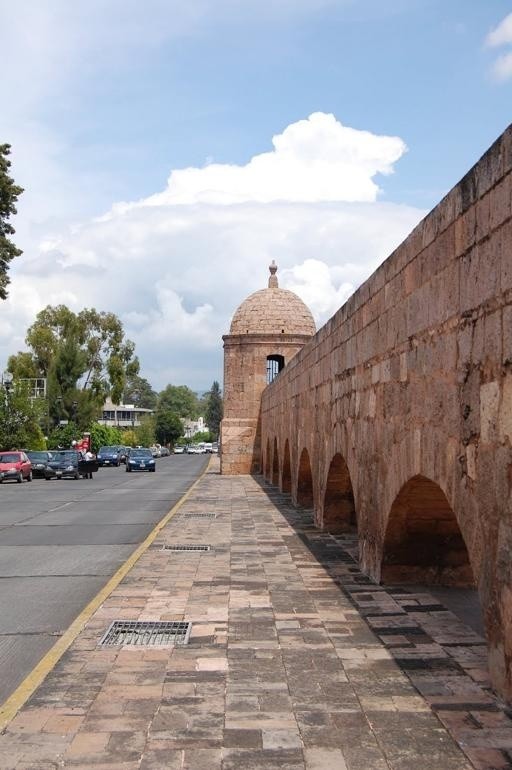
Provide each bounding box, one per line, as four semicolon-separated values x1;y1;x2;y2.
1;371;14;436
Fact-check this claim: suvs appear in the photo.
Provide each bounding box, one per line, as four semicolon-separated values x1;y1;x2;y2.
96;445;121;467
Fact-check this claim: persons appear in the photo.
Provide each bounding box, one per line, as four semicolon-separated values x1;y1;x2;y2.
83;449;96;479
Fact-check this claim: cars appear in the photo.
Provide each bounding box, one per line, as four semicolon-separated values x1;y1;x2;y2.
43;450;99;480
124;449;157;472
15;440;220;463
0;451;33;484
24;451;54;478
